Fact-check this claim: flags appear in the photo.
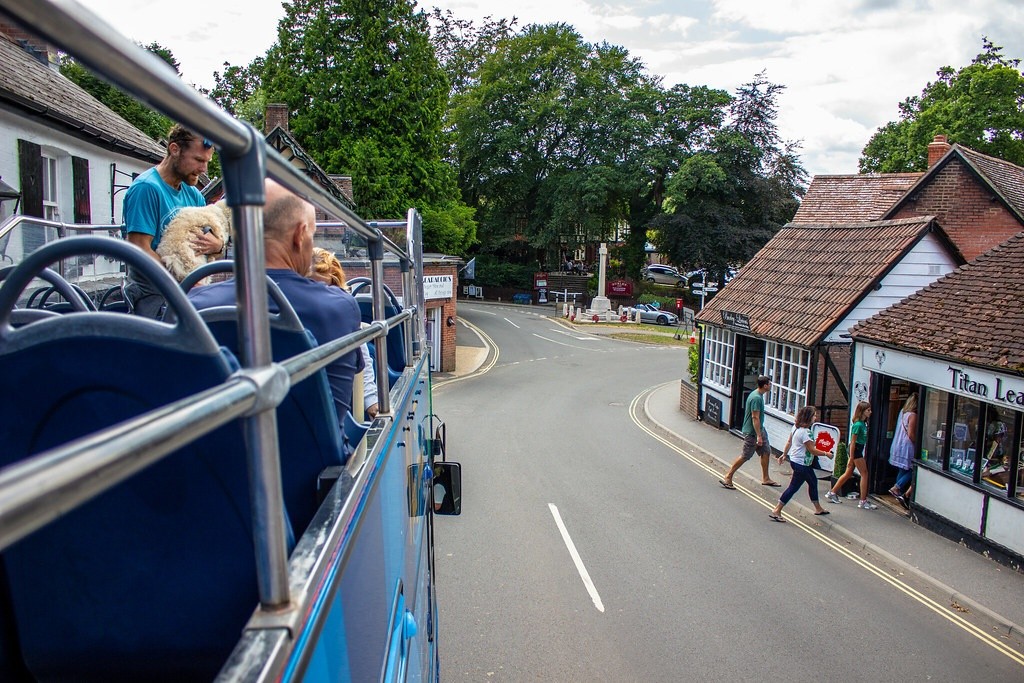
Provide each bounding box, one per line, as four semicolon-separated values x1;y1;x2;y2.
463;262;474;279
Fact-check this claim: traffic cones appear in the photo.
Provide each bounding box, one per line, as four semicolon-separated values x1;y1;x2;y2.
689;327;697;345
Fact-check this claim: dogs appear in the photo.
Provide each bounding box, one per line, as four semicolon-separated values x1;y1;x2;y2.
156;197;233;286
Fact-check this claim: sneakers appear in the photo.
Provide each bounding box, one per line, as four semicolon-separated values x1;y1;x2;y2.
857;500;877;509
824;491;842;504
897;493;910;509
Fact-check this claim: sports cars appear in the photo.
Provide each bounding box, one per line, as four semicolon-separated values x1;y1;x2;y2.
618;303;679;326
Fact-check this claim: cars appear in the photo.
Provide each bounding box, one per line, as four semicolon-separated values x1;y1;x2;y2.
642;263;739;293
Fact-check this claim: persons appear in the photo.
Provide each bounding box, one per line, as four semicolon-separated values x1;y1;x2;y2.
889;392;919;511
718;376;782;488
769;407;834;523
124;120;381;538
561;260;584;274
962;405;1007;460
823;401;878;509
652;299;660;309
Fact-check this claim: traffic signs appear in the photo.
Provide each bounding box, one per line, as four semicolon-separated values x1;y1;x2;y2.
691;282;703;287
704;286;719;292
692;289;708;296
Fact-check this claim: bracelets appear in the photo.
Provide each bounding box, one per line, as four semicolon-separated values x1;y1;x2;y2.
824;452;826;455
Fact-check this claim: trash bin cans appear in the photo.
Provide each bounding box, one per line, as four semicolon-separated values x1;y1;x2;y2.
513;293;533;304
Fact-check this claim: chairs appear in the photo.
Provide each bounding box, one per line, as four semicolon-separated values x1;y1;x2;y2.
0;232;412;683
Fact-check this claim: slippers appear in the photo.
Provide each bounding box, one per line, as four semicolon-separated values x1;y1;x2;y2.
888;489;900;498
815;510;829;515
762;481;781;487
769;515;786;522
719;479;736;490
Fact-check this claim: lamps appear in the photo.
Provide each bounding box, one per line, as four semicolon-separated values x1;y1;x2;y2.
0;175;23;264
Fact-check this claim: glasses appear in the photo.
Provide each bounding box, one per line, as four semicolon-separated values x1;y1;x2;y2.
768;382;772;385
177;137;213;149
